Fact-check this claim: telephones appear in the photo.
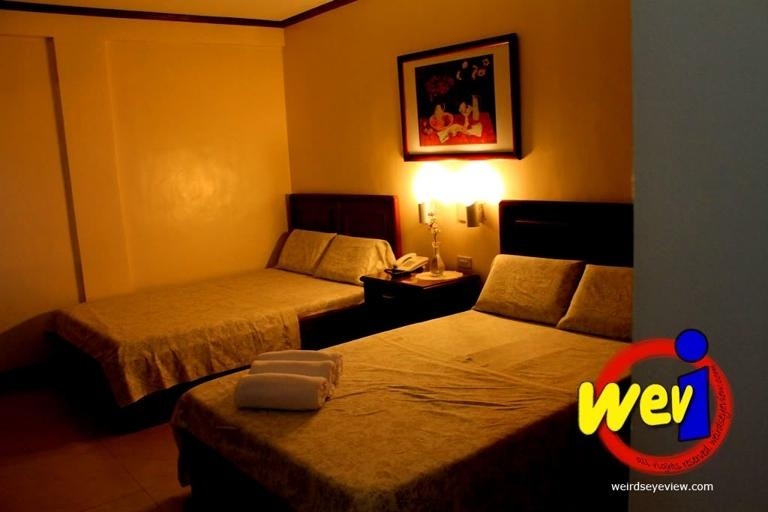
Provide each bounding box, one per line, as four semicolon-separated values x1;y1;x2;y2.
393;252;429;272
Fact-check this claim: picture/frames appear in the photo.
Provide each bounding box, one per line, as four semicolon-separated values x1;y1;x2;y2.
397;33;522;162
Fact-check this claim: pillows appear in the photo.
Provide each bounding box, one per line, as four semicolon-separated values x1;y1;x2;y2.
470;254;633;342
272;228;395;287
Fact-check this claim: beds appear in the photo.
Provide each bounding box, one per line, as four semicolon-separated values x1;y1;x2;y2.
184;201;632;511
67;193;401;433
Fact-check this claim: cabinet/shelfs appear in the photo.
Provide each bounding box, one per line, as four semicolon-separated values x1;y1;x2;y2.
360;271;480;333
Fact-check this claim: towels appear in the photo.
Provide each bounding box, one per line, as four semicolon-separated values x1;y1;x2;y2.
235;350;342;410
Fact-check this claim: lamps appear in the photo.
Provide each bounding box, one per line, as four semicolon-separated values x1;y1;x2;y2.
419;203;434;223
467;202;485;227
